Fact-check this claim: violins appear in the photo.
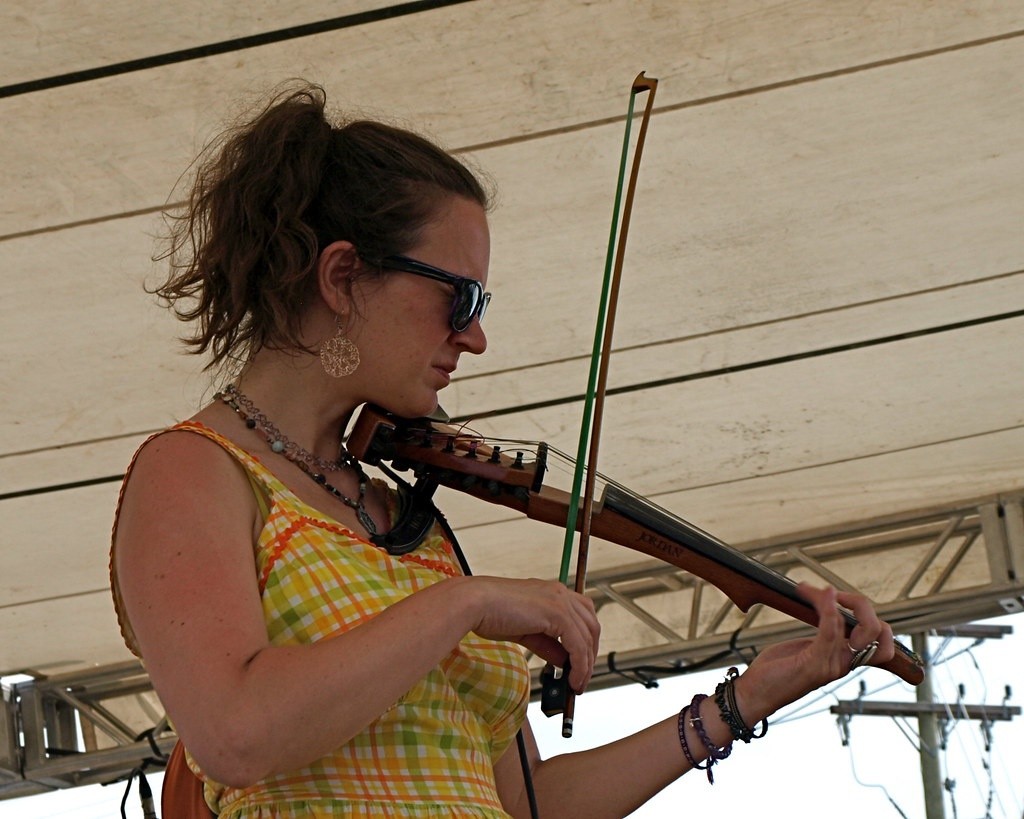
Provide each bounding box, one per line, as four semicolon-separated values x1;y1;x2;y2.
343;403;928;689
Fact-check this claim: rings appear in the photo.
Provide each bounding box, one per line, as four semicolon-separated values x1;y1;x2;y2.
847;641;880;671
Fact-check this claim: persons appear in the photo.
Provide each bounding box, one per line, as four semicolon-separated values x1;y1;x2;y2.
107;77;895;819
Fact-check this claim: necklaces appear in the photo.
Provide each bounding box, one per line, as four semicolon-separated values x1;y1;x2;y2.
213;383;377;543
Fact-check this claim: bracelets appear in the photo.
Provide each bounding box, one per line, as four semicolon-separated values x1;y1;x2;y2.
714;667;768;744
678;694;733;786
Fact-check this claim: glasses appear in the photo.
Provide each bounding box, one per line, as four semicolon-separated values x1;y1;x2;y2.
354;245;492;332
